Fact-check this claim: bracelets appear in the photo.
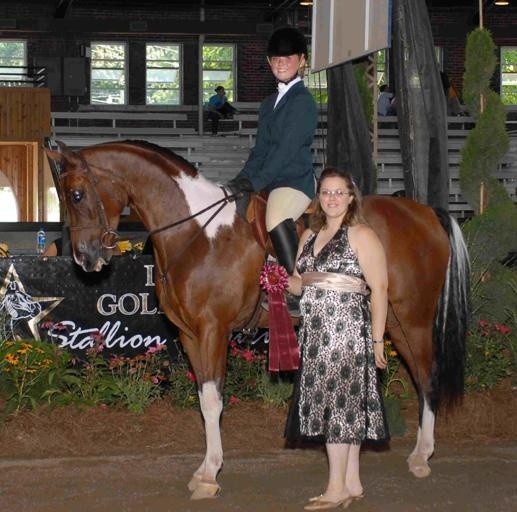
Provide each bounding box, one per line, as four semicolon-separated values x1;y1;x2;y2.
373;339;383;343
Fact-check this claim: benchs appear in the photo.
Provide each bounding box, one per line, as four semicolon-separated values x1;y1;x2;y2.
47;109;204;163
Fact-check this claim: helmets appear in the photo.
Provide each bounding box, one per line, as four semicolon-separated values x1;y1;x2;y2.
268;28;308;61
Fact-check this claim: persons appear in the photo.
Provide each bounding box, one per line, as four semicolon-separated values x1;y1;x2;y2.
377;85;397;130
207;86;241;136
266;166;387;510
43;232;121;258
225;29;318;316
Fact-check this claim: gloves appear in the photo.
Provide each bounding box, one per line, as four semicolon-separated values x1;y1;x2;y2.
226;177;254;192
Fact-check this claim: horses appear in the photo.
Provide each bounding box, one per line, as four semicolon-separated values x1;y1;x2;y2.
39;135;474;501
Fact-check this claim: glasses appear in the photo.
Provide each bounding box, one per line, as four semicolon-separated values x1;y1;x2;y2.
322;190;350;197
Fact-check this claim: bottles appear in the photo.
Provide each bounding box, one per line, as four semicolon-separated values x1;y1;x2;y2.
36;227;46;255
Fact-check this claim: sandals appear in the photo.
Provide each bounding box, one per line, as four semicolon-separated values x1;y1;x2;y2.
303;490;365;510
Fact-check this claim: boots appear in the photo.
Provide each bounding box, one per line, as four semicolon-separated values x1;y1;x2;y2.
260;218;301;318
